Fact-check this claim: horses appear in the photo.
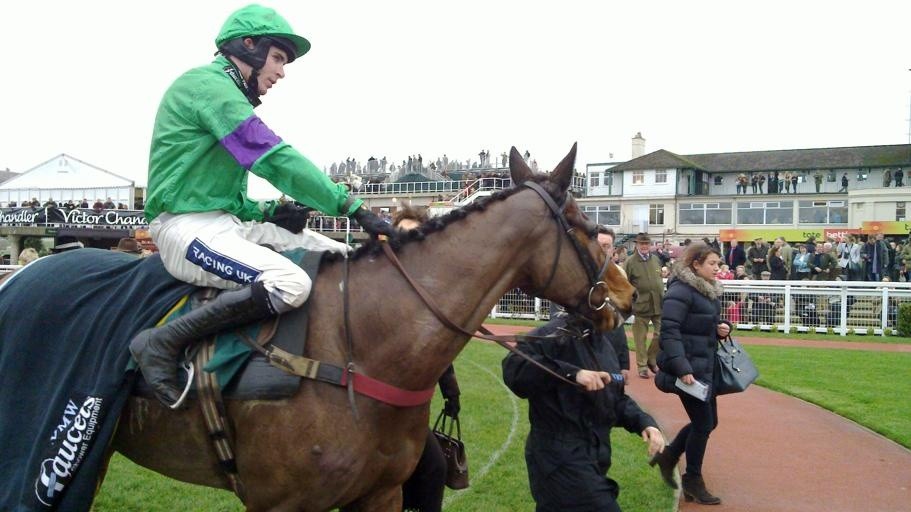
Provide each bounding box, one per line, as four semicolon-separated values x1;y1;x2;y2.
1;140;637;512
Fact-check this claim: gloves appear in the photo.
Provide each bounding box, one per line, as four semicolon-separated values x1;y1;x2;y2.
268;202;310;234
353;209;402;255
442;395;460;419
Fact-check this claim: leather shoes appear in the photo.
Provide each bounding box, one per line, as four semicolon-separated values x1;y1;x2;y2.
639;370;649;379
648;364;660;374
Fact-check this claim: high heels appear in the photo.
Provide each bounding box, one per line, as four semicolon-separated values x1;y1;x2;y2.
682;473;721;505
648;446;679;489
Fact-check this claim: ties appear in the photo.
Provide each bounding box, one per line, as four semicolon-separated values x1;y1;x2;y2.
643;255;648;260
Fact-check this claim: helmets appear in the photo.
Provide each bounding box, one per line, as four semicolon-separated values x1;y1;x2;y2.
219;37;298;69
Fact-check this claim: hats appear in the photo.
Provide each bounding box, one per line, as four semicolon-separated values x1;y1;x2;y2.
632;232;651;243
662;239;672;246
752;235;763;242
214;5;311;60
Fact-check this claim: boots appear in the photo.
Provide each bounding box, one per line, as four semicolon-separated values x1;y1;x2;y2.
128;283;253;413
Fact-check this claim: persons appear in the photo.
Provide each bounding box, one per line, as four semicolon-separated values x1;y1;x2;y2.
304;148;585;205
704;230;910;328
603;325;630;384
626;232;668;379
385;206;461;511
647;242;733;504
734;166;910;195
589;223;628;282
0;197;158;286
130;4;411;412
612;238;690;292
501;307;665;512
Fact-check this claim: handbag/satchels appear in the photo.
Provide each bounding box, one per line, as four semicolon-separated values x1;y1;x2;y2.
432;409;469;489
715;332;758;395
899;274;906;282
847;260;861;273
